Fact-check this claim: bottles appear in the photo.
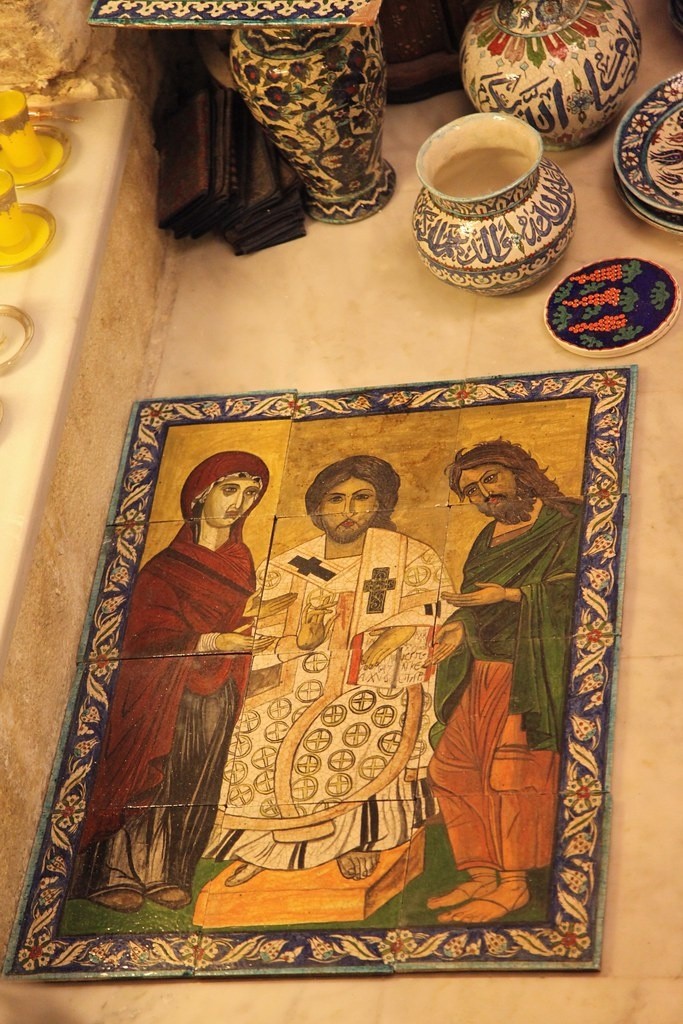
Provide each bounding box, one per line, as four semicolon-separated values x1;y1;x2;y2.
229;0;396;224
410;112;577;296
459;0;642;151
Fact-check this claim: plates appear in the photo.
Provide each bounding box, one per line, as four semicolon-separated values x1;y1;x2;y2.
612;72;683;236
0;125;71;190
0;305;35;373
0;203;56;269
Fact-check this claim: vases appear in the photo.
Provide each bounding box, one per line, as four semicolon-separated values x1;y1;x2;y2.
229;26;398;225
461;0;642;152
412;112;577;298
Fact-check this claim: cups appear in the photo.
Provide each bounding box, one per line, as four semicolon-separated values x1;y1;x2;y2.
0;90;46;174
0;169;32;255
